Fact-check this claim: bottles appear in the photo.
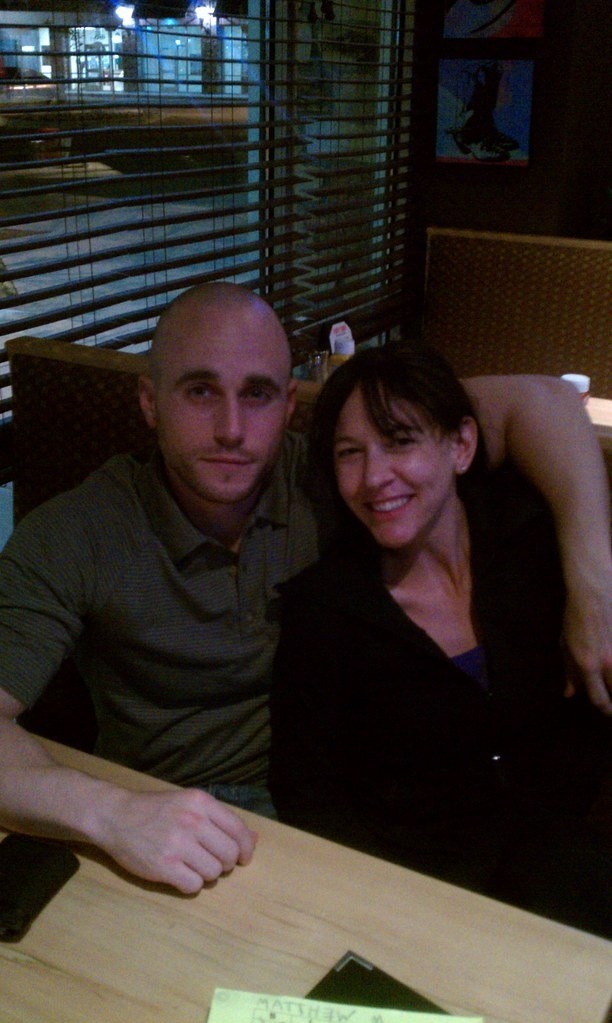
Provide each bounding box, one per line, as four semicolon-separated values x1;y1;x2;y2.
560;373;590;405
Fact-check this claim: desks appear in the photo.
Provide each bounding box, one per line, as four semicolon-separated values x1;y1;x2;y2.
0;732;612;1023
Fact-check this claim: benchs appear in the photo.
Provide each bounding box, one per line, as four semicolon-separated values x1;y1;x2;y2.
425;226;612;413
4;336;611;533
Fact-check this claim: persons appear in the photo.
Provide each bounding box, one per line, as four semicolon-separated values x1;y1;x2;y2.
266;342;612;940
0;283;612;898
